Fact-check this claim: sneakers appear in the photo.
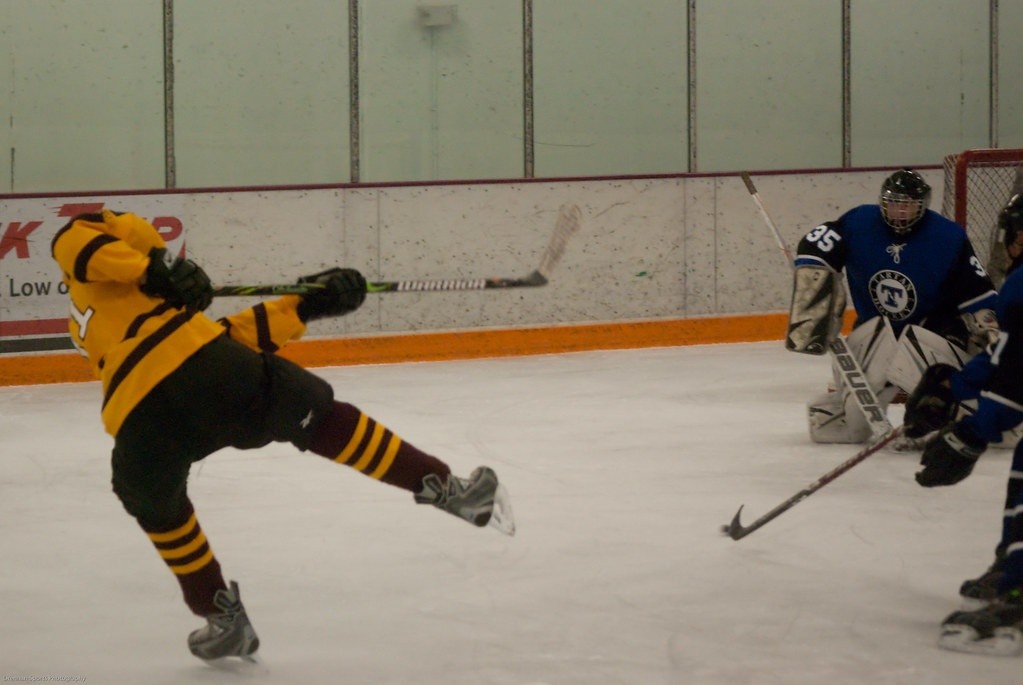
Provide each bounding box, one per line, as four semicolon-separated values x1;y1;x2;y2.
188;580;259;672
940;593;1023;656
959;546;1009;612
415;467;515;535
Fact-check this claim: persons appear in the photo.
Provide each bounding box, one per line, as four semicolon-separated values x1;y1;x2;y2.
49;210;516;680
784;169;1023;657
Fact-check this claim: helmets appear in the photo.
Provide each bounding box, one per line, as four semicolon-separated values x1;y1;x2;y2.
999;193;1023;235
880;170;929;231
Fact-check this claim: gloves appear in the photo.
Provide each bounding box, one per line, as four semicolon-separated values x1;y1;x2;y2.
142;247;213;315
916;416;990;487
297;266;367;324
904;364;961;439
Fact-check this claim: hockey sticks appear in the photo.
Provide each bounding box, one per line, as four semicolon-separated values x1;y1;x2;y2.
721;422;920;542
137;202;581;297
739;170;934;453
